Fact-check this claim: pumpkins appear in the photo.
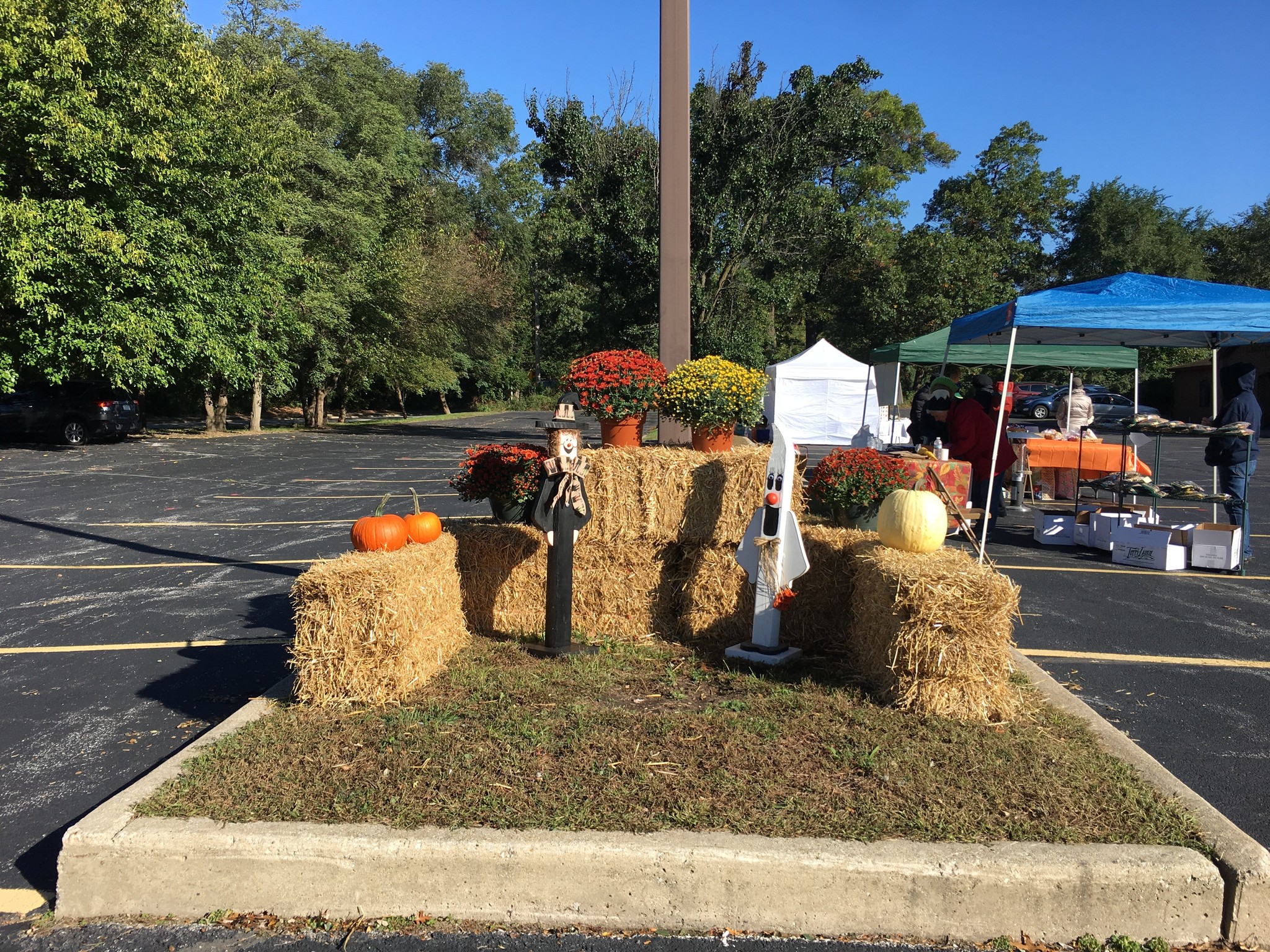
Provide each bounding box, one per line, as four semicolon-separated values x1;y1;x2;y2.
877;477;948;554
402;487;441;544
350;494;408;553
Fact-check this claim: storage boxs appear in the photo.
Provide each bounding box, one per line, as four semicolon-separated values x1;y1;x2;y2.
1032;500;1243;572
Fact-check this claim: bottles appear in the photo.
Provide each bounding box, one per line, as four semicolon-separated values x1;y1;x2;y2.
933;437;942;460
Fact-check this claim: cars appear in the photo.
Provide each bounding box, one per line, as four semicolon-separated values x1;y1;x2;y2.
1012;381;1058;413
0;377;145;447
1084;391;1160;426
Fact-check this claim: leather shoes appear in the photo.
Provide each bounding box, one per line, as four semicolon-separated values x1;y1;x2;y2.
1244;558;1248;566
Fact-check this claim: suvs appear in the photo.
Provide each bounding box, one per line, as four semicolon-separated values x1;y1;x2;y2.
1015;384;1113;420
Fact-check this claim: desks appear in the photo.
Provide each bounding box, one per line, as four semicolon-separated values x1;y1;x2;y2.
1023;435;1138;507
878;417;912;449
1006;430;1046;440
888;450;973;541
1115;427;1252;577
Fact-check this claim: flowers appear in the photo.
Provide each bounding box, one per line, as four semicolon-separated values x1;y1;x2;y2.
654;353;773;441
803;444;915;510
442;440;551;506
555;347;669;424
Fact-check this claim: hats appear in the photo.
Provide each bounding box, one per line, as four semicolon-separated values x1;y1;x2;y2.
1068;377;1084;389
926;390;951;411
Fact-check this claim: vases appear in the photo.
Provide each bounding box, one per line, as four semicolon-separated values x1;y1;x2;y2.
486;492;536;526
596;410;647;450
831;496;884;536
688;419;737;455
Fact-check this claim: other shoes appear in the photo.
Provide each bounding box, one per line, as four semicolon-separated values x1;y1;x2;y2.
966;530;992;542
959;529;974;537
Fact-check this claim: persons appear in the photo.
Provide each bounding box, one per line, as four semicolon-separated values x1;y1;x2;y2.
907;362;1018;542
1203;358;1262;564
1055;377;1095;433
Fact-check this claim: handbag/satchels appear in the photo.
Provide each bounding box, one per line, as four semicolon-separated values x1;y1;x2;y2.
1030;466;1082;501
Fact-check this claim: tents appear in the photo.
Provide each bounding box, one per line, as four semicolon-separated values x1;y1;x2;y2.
862;323;1139;507
938;272;1270;565
763;339;913;446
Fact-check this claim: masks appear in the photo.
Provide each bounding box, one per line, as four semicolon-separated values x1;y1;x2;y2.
970;375;993;389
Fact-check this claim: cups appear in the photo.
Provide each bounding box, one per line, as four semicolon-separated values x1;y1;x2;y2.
940;448;949;461
